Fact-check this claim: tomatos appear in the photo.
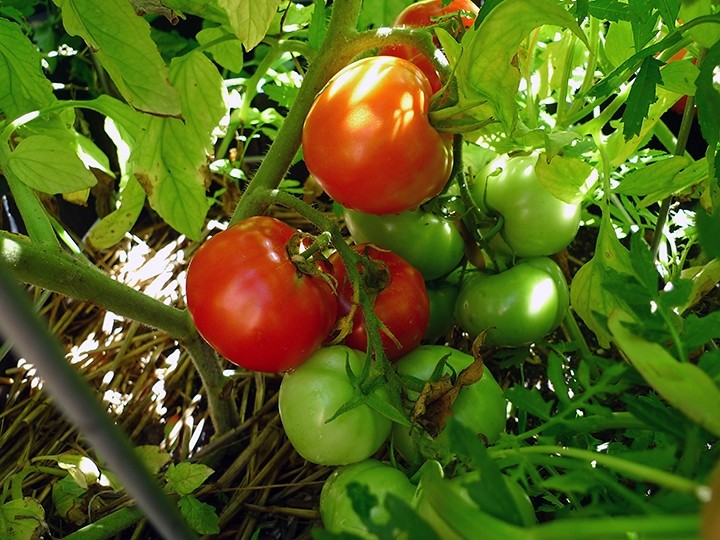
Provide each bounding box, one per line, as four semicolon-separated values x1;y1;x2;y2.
184;0;720;540
